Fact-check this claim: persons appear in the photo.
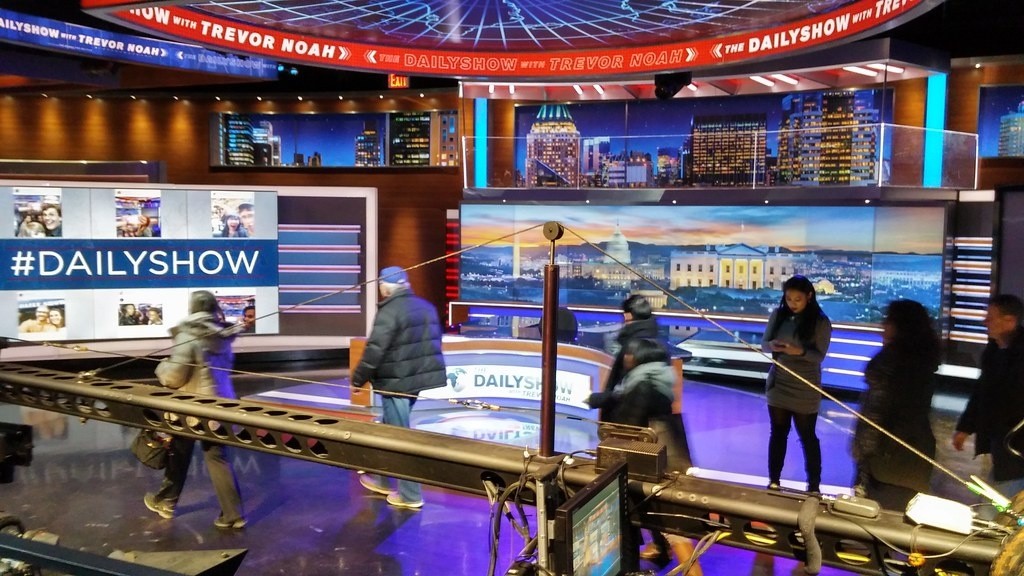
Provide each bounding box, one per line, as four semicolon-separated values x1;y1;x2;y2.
761;275;832;490
599;295;658;423
145;291;247;526
18;206;61;237
587;339;675;563
212;203;254;238
20;305;66;332
119;304;162;325
853;300;940;509
952;296;1024;522
242;306;255;332
350;267;447;508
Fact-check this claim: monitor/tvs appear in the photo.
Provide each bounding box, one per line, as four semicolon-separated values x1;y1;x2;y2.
554;460;633;576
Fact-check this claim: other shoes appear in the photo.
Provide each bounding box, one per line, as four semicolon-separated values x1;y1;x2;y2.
360;473;399;494
386;492;426;508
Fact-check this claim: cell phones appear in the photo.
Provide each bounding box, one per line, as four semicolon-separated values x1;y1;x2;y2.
775;343;786;348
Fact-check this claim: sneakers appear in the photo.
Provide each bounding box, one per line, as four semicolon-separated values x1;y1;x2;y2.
213;516;249;529
144;490;177;519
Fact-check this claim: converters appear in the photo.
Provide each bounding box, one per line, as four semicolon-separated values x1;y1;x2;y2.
595;438;668;483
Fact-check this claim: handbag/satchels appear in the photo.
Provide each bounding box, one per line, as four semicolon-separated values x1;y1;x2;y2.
130;427;176;470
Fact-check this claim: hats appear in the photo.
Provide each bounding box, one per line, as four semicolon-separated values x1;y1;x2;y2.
524;306;579;334
379;265;407;285
236;197;254;208
621;294;652;319
